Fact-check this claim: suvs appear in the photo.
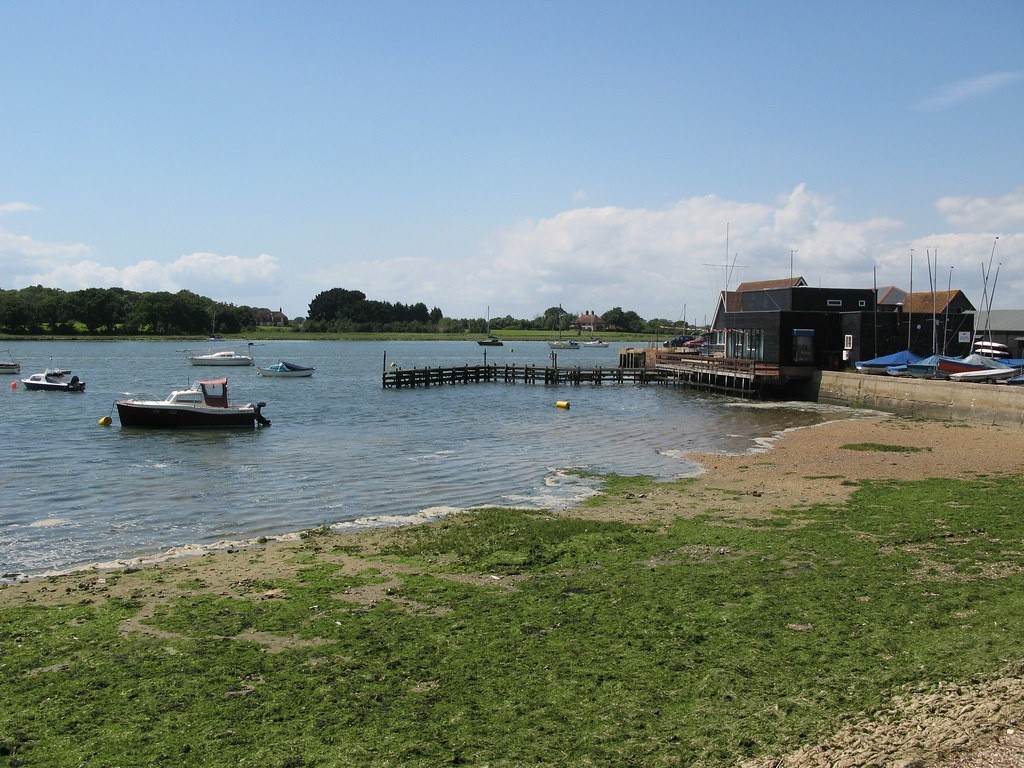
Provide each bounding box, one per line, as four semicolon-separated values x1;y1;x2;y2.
663;335;695;348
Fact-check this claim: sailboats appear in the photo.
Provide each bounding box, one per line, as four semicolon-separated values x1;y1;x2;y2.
854;236;1024;386
476;306;504;346
548;304;580;349
581;312;610;347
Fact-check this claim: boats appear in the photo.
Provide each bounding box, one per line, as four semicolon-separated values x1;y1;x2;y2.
258;358;316;377
0;349;21;375
186;342;255;366
115;378;270;428
22;368;86;393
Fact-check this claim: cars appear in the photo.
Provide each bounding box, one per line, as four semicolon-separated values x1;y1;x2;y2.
686;334;708;348
